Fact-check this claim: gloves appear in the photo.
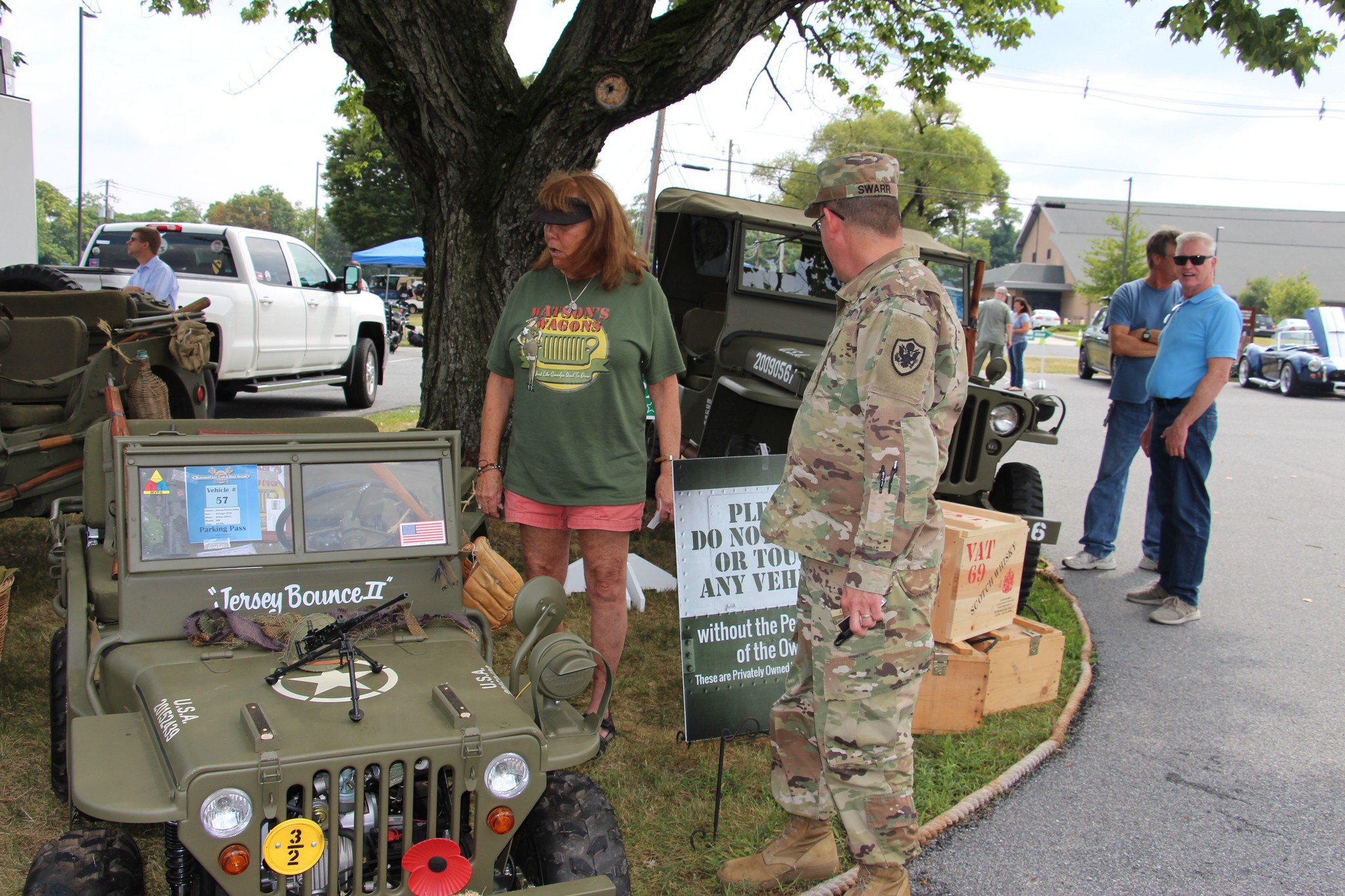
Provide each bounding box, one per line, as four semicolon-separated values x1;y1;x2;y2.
459;536;523;633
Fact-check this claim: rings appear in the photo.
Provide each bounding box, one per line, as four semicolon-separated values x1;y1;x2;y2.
861;614;871;619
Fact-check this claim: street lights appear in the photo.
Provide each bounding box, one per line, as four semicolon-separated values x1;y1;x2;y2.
76;6;98;268
1212;226;1225;283
1120;178;1132;286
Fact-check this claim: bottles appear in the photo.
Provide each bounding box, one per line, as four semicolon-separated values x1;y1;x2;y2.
128;349;171;420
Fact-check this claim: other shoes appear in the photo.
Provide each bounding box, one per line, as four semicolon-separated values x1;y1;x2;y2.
1003;386;1022;391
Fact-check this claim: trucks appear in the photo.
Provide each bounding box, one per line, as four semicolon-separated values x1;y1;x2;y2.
646;185;1066;613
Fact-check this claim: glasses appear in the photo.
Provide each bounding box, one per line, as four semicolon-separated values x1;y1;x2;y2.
812;209;844;233
130;237;144;242
1173;254;1214;265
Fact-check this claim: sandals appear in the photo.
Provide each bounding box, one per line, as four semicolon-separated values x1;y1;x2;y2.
582;708;617;760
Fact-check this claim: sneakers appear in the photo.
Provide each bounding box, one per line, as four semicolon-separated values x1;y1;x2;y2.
844;863;910;896
1126;580;1174;604
1138;555;1158;571
717;813;840;891
1149;596;1200;624
1062;551;1117;570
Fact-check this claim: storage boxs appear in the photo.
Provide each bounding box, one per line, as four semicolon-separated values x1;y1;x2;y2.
911;499;1067;735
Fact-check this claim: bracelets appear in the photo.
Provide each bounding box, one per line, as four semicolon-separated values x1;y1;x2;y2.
653;454;679;462
475;460;503;476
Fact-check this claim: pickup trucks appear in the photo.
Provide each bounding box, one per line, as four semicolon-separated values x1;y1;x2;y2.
39;221;392;419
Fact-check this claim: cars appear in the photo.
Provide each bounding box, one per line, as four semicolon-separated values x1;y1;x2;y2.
1270;317;1312;340
1253;314;1277;338
1234;305;1345;401
367;273;425;314
1030;308;1061;330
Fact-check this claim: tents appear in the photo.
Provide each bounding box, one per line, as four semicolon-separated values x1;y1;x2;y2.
351;236;427;301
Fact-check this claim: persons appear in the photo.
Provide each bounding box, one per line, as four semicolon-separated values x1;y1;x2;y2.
971;286;1013;385
1004;297;1031;391
413;280;426;298
1062;228;1184;569
124;227;179;312
1127;231;1244;625
474;171;685;761
718;149;967;896
346;260;369;292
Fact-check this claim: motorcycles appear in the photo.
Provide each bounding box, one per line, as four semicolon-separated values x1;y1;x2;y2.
385;307;410;354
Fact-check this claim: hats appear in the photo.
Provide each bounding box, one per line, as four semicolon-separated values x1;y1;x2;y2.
994;286;1011;297
804;152;899;218
525;197;593;225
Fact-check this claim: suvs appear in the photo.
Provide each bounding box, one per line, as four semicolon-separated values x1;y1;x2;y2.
0;262;221;522
1077;296;1118;382
42;415;637;896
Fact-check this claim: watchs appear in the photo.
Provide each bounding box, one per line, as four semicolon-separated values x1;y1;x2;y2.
1142;328;1152;342
